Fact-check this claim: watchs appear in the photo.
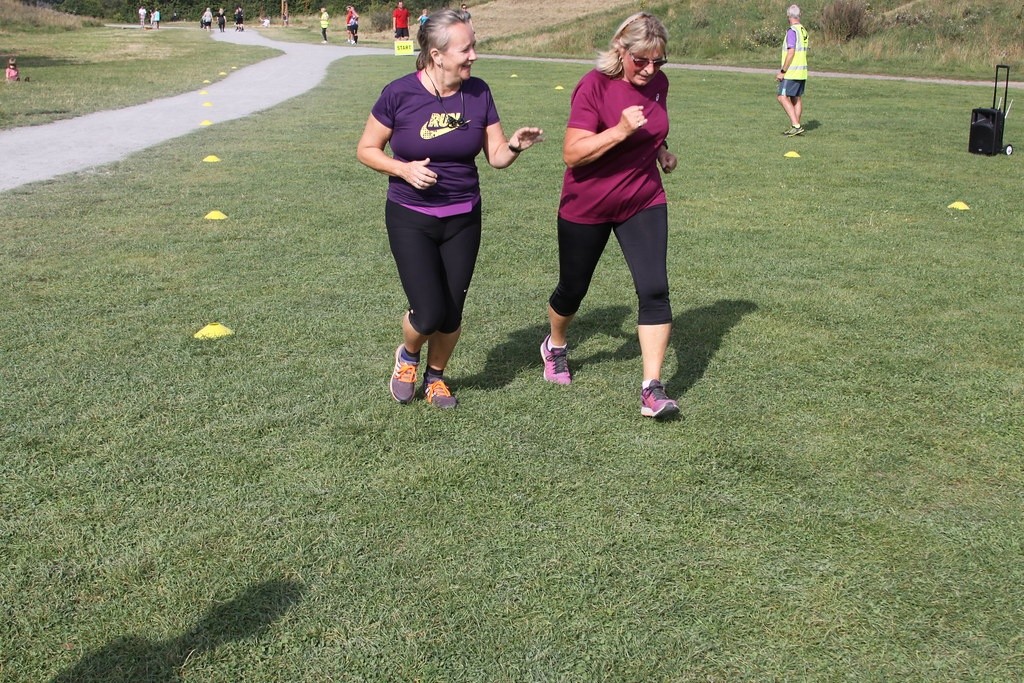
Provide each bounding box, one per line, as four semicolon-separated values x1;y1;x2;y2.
781;69;786;73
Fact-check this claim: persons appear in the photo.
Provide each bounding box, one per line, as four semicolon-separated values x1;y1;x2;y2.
138;5;160;30
416;9;432;25
356;8;545;408
392;0;409;41
461;4;473;30
775;5;809;137
202;7;359;45
540;13;681;421
5;58;19;82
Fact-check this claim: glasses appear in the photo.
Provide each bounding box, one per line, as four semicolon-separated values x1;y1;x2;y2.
626;46;668;66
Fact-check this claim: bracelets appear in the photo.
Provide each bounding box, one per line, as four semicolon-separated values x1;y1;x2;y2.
508;142;523;154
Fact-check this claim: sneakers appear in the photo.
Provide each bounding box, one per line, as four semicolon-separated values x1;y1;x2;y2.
640;379;679;417
787;124;804;137
423;372;457;408
783;125;792;135
540;333;571;386
390;343;420;402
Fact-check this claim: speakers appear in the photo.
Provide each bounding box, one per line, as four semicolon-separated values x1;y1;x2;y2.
968;107;1005;157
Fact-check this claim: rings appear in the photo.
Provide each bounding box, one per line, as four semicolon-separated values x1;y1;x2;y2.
417;179;419;185
638;123;641;127
638;118;639;122
